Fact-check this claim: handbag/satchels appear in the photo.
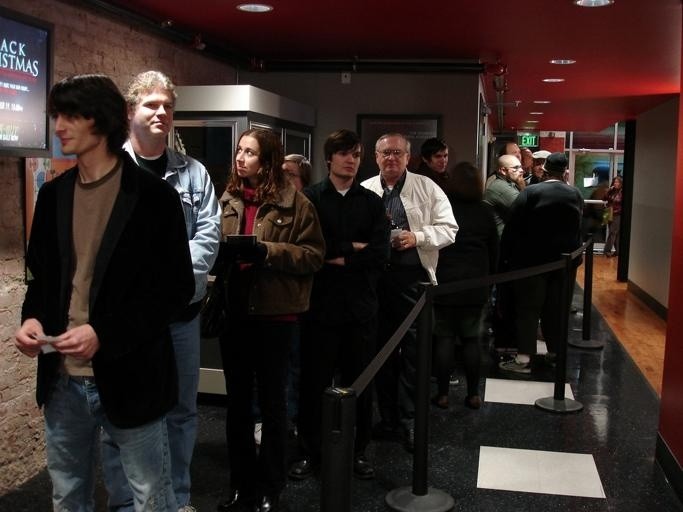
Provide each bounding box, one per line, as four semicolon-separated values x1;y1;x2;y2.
602;206;613;225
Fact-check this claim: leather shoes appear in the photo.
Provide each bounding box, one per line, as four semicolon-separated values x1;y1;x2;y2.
219;487;274;511
354;460;376;479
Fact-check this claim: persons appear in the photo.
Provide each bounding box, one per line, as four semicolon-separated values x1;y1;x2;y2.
95;69;222;512
280;129;500;486
480;139;582;373
216;124;326;512
601;177;625;257
14;73;194;512
589;171;611;244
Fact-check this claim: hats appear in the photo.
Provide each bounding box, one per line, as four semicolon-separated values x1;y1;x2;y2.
531;150;552;159
542;152;568;176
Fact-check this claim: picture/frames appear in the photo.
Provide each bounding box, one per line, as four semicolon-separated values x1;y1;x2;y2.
355;113;444;186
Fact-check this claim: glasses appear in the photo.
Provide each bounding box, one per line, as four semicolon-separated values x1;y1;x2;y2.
376;147;405;155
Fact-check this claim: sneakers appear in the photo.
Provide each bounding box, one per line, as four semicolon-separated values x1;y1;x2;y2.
431;397;449;409
499;361;531;373
465;396;481;408
